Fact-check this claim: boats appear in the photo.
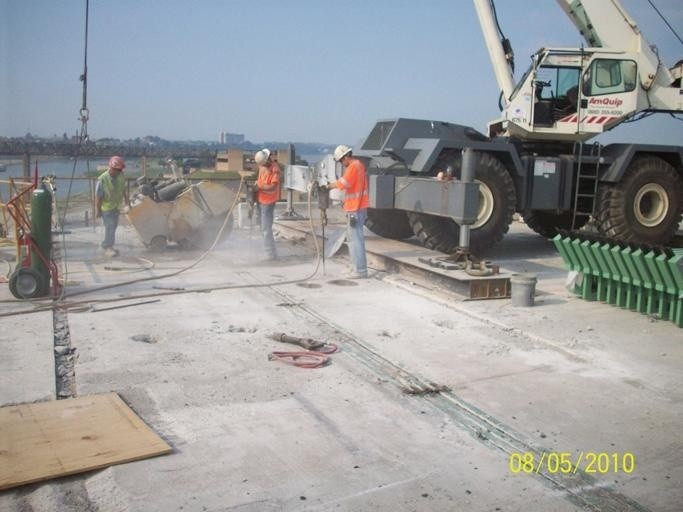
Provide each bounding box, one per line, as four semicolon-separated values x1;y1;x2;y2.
80;165;182;181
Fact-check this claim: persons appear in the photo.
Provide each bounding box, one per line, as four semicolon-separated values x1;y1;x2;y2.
95;156;129;257
326;145;368;278
254;148;279;260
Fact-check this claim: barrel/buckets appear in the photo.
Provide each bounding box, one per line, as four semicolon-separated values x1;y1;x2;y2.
239;202;256;225
510;273;537;306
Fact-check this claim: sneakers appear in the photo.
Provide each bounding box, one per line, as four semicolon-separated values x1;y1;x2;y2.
260;254;277;261
103;248;119;258
347;272;368;279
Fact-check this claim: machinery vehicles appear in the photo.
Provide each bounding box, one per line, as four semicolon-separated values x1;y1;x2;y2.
351;0;682;264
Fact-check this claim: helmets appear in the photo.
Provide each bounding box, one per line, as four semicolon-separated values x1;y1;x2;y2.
254;148;271;164
109;156;126;170
334;145;353;162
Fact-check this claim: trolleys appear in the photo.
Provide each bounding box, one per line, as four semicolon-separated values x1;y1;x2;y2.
5;191;45;301
